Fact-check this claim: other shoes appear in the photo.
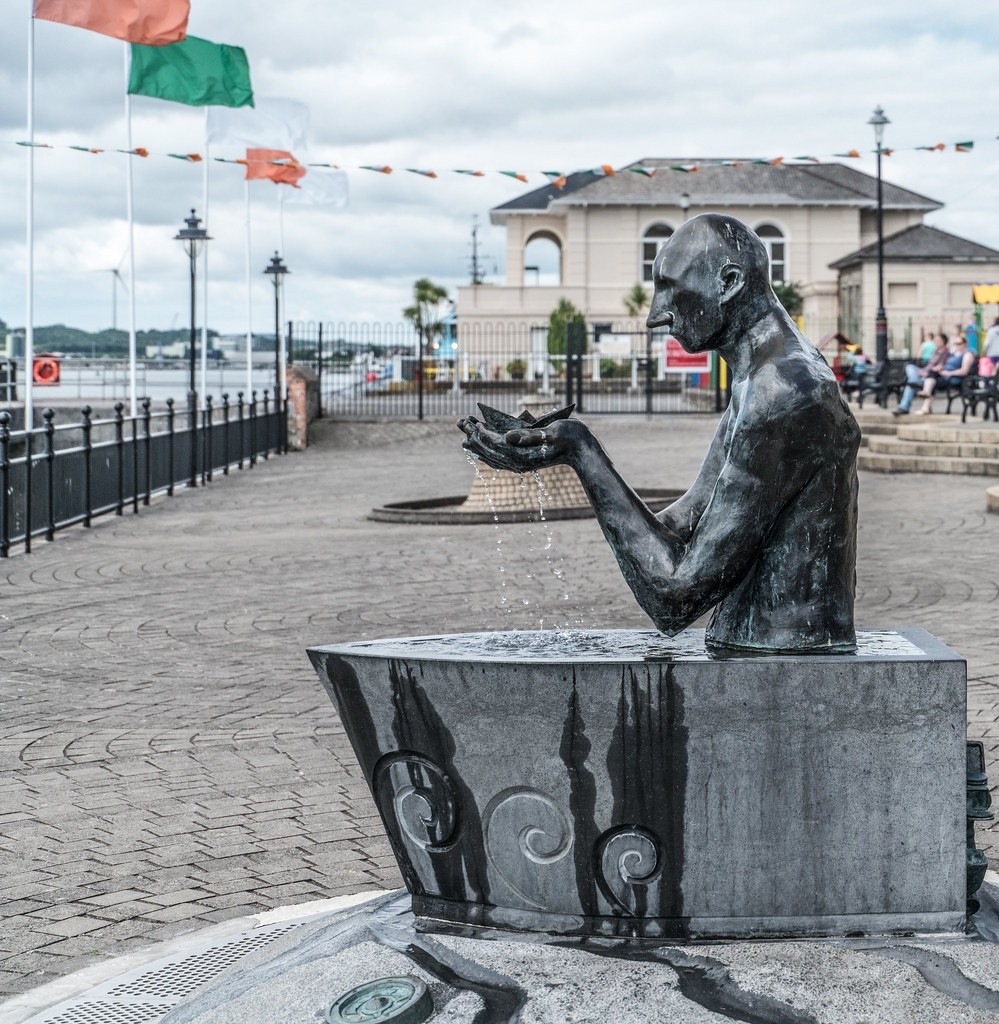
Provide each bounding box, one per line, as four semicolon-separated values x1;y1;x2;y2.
916;390;930;398
892;408;909;415
916;408;932;415
908;381;923;391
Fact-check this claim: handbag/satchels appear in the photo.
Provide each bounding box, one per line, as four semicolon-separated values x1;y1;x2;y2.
978;357;998;378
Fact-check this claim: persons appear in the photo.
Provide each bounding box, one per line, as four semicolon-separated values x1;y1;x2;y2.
454;213;862;658
890;314;999;416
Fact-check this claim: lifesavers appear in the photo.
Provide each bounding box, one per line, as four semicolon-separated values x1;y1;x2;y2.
33;359;59;383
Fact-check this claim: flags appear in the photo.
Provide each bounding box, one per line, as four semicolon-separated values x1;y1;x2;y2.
246;147;305;189
18;140;974;191
127;29;255;110
32;0;192;47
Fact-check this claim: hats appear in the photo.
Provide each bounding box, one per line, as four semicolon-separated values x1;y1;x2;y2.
953;337;967;345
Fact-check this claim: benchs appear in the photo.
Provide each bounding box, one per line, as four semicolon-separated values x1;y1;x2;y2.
838;357;999;424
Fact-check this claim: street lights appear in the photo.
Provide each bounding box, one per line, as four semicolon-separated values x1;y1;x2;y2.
172;208;215;425
865;104;894;412
263;250;290;413
